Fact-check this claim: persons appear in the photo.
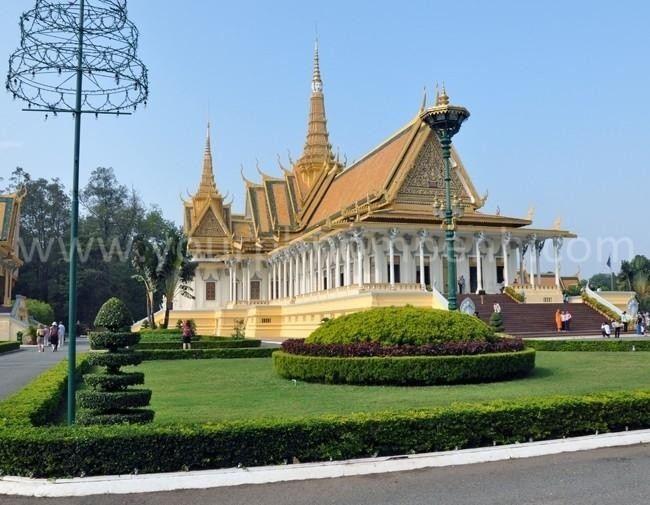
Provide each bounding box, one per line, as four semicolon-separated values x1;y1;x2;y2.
555;309;562;333
37;320;67;352
600;310;649;338
457;274;465;294
514;278;517;283
561;311;566;331
179;318;193;349
565;310;572;331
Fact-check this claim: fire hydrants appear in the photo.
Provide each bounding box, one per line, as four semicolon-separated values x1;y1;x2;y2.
3;0;150;428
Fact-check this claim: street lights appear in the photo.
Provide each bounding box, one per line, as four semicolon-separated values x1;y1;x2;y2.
418;81;473;311
